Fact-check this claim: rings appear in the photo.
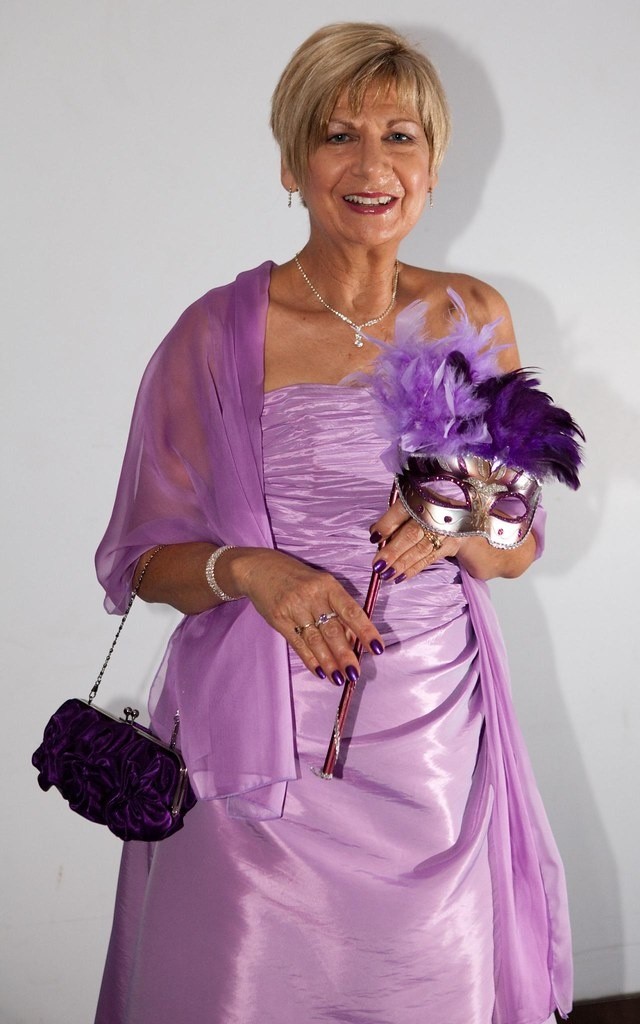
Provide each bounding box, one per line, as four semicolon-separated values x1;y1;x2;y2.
315;612;337;628
426;533;441;549
294;622;314;634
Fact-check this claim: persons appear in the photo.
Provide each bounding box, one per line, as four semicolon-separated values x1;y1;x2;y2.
93;23;575;1024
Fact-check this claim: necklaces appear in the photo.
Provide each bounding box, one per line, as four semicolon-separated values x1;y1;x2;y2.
295;254;399;347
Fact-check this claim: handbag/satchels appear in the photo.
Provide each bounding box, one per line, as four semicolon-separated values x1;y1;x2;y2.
32;544;197;842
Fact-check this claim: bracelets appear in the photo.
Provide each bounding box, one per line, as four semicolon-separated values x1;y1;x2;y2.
205;545;242;601
